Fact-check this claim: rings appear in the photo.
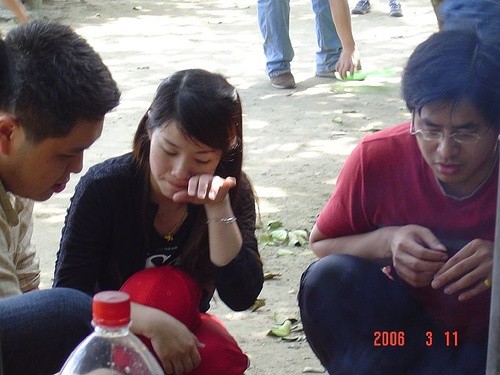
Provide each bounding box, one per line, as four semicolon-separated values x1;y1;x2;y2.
484;280;491;287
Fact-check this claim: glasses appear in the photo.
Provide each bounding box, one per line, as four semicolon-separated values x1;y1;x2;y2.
409;109;492;145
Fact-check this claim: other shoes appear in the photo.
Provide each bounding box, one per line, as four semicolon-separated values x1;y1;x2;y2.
315;70;336;77
270;72;296;89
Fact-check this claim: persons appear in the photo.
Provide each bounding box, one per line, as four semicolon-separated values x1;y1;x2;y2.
297;29;500;375
255;0;362;89
352;0;404;17
0;17;112;375
430;0;500;49
52;70;266;375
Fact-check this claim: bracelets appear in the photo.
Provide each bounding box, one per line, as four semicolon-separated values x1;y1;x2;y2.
207;215;236;225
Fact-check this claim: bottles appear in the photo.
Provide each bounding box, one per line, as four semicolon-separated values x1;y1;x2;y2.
56;291;166;375
